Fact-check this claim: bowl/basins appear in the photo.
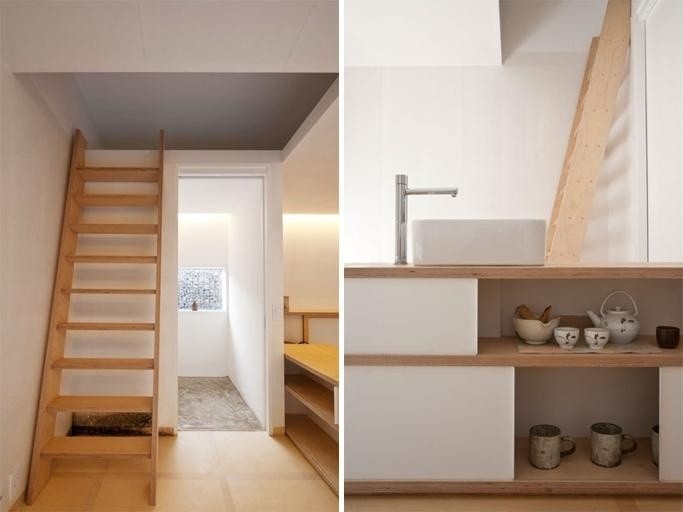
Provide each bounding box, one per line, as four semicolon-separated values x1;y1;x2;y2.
513;315;561;346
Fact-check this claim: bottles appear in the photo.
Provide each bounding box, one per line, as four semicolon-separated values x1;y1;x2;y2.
191;300;198;311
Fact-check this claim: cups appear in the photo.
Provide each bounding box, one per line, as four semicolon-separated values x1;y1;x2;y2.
656;326;681;348
554;327;580;348
590;422;637;468
651;425;660;466
529;424;576;470
584;327;611;348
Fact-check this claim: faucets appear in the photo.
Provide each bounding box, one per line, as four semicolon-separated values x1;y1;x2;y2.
395;174;457;265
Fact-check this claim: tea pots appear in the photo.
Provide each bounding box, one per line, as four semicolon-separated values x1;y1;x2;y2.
586;291;640;346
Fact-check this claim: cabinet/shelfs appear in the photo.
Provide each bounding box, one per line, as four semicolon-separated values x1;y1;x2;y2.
343;274;683;496
282;345;340;499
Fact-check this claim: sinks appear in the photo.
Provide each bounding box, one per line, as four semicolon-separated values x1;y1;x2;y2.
408;217;546;265
